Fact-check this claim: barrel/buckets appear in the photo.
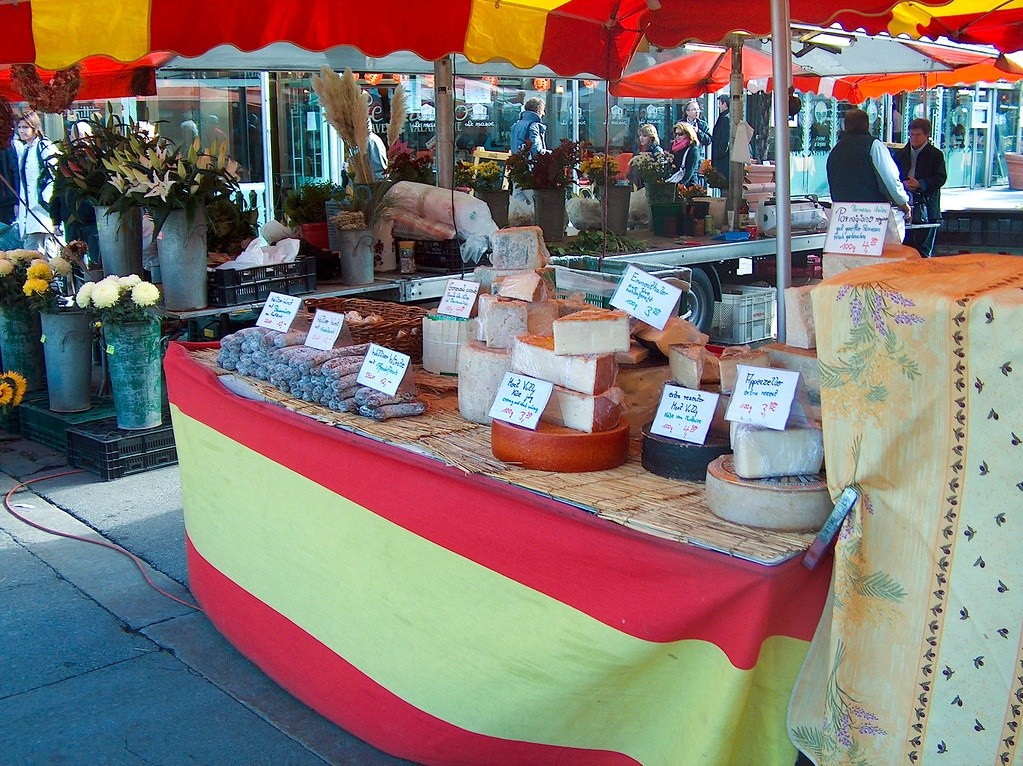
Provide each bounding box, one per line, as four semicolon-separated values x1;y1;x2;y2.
693;197;729;230
648;203;684;236
688;202;711;236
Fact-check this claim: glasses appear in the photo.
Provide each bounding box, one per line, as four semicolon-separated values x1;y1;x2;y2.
687;108;700;112
674;132;685;136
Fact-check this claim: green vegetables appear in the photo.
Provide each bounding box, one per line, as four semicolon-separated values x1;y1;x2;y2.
547;227;657;256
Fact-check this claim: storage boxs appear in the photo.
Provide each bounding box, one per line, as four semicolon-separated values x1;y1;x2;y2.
705;284;777;344
187;313;258;342
19;394;117;450
753;255;822;279
0;390;49;435
208;255;316;307
546;254;691;314
65;413;178;480
394;237;493;275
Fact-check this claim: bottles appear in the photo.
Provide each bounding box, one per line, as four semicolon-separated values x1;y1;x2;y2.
663;199;761;237
398;240;416;274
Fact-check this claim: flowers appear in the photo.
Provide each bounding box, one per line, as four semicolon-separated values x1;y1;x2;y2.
629;150;676;182
0;370;28;414
676;182;709;204
0;239;168;341
1;63;593;229
699;158;753;193
579;153;620;188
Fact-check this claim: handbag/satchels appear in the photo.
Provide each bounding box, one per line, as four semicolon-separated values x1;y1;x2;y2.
666;167;686;184
913;194;928;224
36;141;52;213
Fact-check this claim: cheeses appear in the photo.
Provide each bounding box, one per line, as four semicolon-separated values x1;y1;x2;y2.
423;266;834;534
822;244;921;279
491;226;550;270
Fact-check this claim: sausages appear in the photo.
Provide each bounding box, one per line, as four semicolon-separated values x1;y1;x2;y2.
217;327;428;421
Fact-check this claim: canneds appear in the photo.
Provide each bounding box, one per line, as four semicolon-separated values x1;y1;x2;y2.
704;215;715;236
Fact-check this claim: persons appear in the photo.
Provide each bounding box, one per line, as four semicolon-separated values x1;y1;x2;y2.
201;114;227;153
629;104;658;141
711;94;730;181
826;109;911;243
636;124;663;156
15;111;62;258
894;118;947;258
678;100;711;153
952;124;965;148
609;94;629;147
346;120;389;186
178;120;199;158
510;97;552;196
49;120;103;266
891;102;902;142
0;141;23;252
662;121;701;187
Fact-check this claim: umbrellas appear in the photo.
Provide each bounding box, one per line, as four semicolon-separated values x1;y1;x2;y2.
0;0;1023;343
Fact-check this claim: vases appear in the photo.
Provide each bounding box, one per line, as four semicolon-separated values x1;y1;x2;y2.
1005;151;1023;190
743;163;776;212
